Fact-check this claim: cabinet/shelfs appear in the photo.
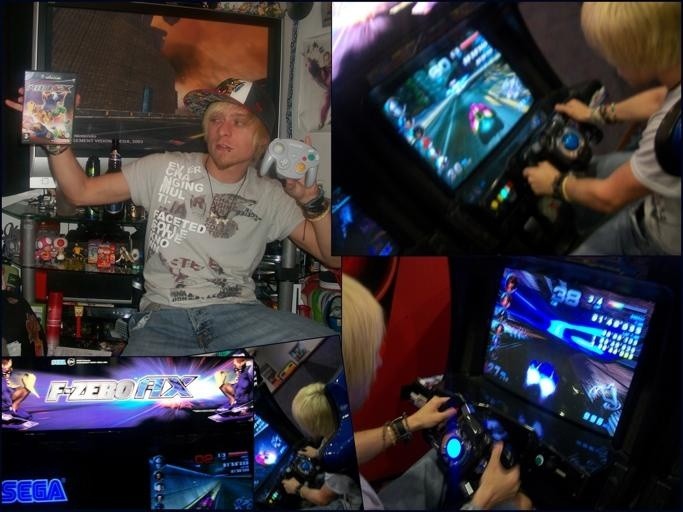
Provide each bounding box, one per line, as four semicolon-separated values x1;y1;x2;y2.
1;190;297;316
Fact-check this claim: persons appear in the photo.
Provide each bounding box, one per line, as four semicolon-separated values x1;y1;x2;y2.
306;51;332;129
4;78;342;357
281;381;362;512
345;275;534;512
523;1;681;255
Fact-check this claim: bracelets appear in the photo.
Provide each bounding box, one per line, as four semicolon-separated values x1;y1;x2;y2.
592;100;616;129
554;172;574;202
46;144;68;155
295;482;306;499
459;503;483;511
384;417;414;447
297;188;331;222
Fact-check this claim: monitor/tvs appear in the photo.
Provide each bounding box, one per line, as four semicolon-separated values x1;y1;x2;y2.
380;27;533;189
0;429;254;511
480;266;657;441
254;405;289;495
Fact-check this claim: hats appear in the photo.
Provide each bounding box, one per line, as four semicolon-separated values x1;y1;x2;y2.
182;77;276;140
